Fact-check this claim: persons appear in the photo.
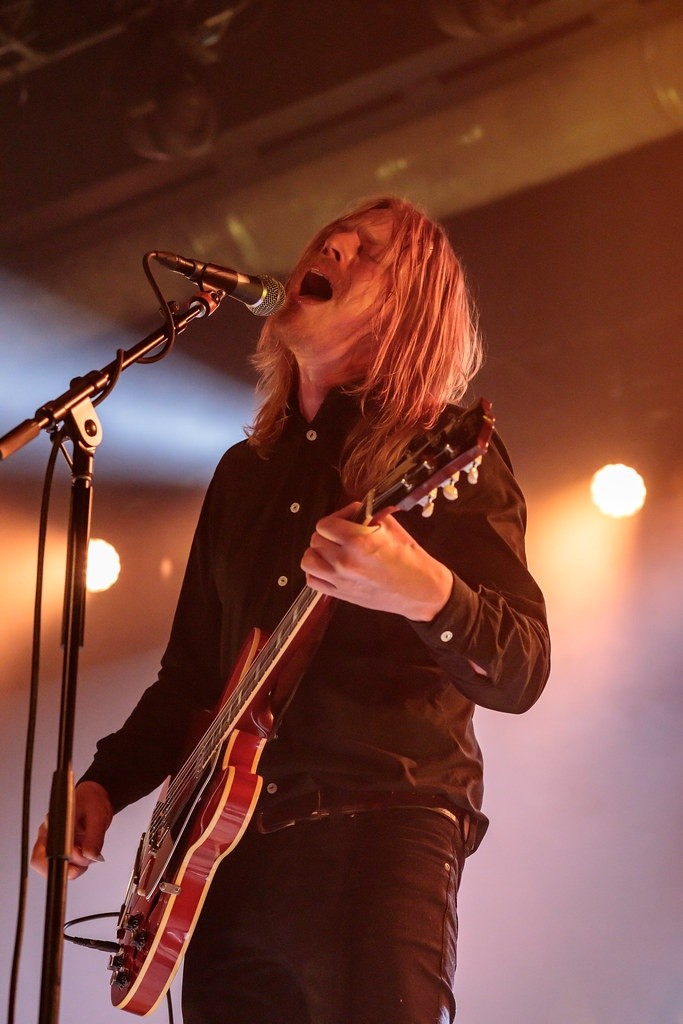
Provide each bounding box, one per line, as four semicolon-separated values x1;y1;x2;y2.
32;197;552;1024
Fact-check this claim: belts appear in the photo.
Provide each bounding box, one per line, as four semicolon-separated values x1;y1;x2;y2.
244;790;471;842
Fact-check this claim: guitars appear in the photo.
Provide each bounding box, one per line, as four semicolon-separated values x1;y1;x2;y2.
105;396;498;1016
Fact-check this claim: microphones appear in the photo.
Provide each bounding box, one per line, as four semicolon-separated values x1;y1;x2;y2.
151;249;286;319
